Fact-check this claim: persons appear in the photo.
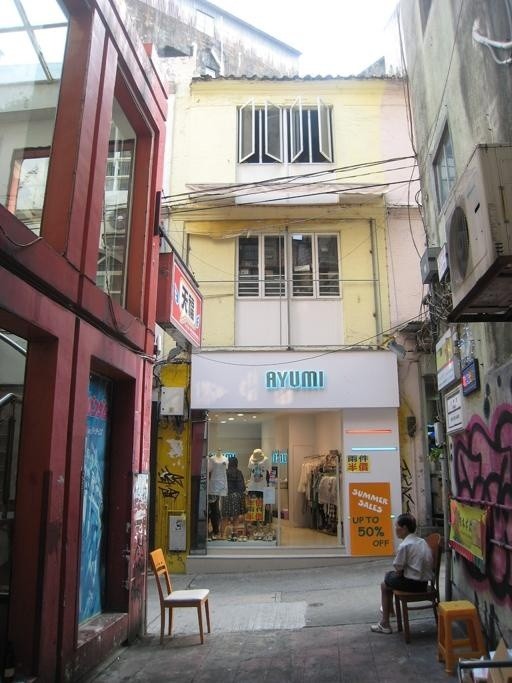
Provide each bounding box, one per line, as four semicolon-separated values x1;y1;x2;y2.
218;456;246;539
207;447;229;497
245;448;271;531
369;512;435;635
207;493;221;537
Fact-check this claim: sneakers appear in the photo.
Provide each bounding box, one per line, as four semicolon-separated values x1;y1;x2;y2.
371;623;392;634
380;605;395;617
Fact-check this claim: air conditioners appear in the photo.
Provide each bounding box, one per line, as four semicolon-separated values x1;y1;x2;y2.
435;139;511;324
101;202;129;243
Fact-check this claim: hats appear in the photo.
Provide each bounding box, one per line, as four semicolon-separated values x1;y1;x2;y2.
251;448;266;463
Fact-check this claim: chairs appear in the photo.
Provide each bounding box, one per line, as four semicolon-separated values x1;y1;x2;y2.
149;547;211;644
394;532;442;644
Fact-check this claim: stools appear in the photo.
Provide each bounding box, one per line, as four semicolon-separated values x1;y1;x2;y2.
437;599;487;673
